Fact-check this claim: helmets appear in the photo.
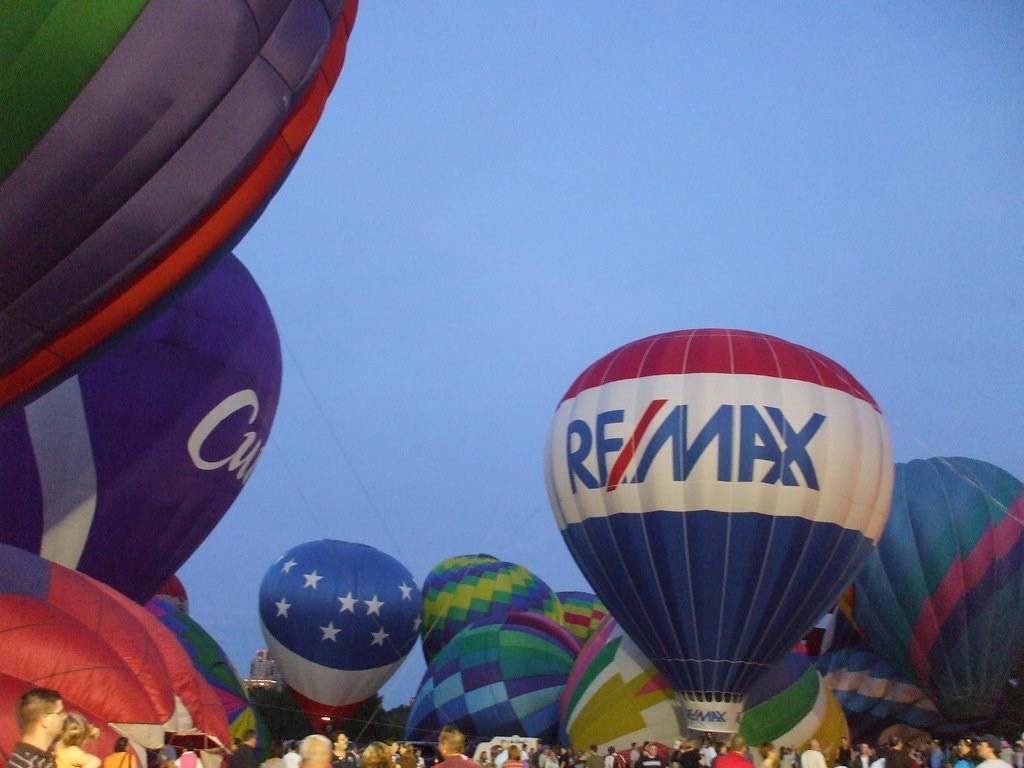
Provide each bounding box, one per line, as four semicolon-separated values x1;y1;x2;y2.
1015;739;1024;747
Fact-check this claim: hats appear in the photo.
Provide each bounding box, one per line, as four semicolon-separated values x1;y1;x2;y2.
975;733;1002;752
541;744;551;750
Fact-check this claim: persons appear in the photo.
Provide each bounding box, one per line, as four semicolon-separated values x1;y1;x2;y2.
0;686;1024;768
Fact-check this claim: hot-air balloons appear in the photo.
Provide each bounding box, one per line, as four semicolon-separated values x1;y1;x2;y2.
402;329;1024;768
259;539;427;737
0;1;362;768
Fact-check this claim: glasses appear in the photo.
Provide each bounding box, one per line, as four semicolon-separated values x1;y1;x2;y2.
44;708;67;716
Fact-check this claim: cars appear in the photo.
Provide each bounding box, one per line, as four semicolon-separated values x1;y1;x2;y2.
396;741;445;767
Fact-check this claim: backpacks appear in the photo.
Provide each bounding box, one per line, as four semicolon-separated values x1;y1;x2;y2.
610;753;626;768
